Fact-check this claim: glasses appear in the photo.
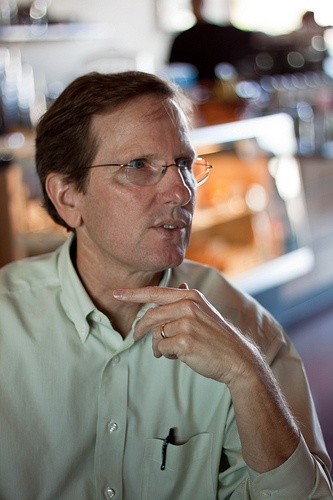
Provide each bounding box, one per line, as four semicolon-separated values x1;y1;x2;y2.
57;157;212;187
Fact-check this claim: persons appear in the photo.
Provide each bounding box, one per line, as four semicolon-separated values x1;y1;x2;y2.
0;71;332;500
168;0;241;83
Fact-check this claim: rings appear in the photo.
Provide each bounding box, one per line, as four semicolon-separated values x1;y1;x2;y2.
160;323;167;339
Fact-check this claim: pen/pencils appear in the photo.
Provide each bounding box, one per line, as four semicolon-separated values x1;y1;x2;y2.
161;428;176;470
